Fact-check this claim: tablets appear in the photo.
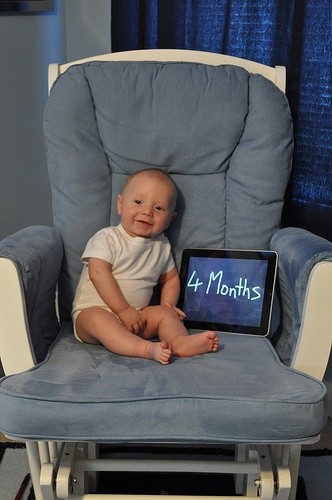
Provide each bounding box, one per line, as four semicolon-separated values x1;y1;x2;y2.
179;248;278;337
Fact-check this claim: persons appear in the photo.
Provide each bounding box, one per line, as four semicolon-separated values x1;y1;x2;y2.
70;166;221;366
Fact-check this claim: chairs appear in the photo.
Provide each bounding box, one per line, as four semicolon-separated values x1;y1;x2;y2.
0;49;332;500
